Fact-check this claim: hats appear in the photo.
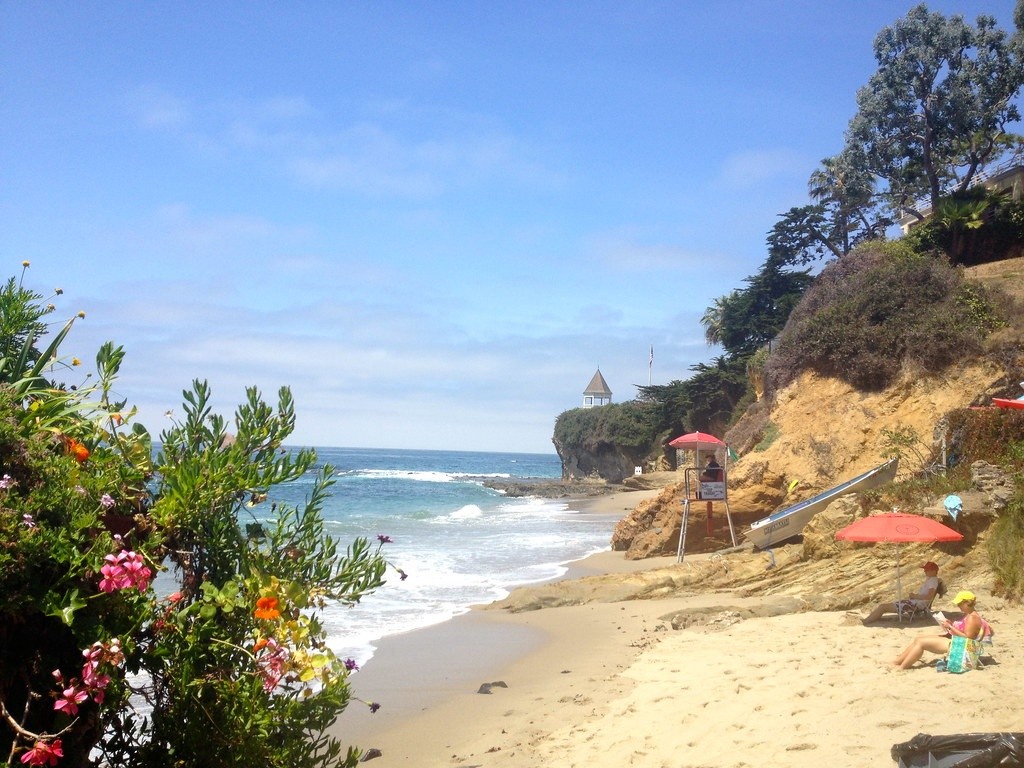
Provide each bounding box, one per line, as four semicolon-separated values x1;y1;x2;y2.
705;452;714;458
921;562;939;573
951;590;975;604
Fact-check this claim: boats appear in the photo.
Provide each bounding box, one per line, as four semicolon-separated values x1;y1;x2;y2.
741;456;898;550
991;398;1024;409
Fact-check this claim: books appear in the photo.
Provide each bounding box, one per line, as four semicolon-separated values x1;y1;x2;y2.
933;611;947;632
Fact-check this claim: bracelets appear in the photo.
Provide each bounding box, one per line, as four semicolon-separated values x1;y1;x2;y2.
949;626;952;630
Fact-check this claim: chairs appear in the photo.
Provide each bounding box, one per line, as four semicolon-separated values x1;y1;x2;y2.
908;578;949;624
695;466;723;499
943;618;997;668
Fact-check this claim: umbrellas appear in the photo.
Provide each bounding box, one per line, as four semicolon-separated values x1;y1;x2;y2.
836;507;965;622
669;431;727;500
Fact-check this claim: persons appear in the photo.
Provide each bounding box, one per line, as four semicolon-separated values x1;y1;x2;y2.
894;591;982;669
695;454;720;482
864;562;939;623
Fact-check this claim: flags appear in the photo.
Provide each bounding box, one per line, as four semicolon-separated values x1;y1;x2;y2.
649;346;653;368
729;448;738;461
